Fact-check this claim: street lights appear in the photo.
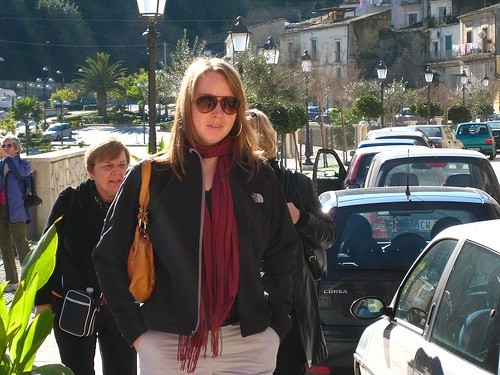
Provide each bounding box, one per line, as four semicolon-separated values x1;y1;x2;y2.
376;60;391;128
460;70;469;105
229;15;255;82
425;66;434;125
481;74;490;121
299;50;315;166
16;67;71;156
137;0;168;154
260;36;282;97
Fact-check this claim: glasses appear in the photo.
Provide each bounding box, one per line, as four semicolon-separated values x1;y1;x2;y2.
193;93;242;115
246;112;258;118
2;144;16;148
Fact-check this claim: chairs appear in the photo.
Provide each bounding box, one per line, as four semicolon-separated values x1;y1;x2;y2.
475;127;488;135
430;216;465;240
386;233;427;263
444;174;472;187
341;214;384;264
461;128;472;136
457;266;500;360
389;171;418;187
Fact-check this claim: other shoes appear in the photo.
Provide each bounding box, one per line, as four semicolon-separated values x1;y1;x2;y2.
4;282;19;291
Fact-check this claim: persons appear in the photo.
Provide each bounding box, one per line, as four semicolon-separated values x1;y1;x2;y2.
486;265;500;330
242;110;338;375
32;140;144;375
92;56;300;375
0;136;43;291
340;215;384;261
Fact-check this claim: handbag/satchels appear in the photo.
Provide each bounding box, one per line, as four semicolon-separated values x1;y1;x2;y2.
301;237;328;280
58;289;100;339
127;159;157;303
24;173;42;208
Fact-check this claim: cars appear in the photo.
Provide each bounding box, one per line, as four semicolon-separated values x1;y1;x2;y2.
291;184;500;375
307;106;327;122
165;116;172;121
43;122;72;141
311;119;500;198
347;218;499;375
398;108;418;121
315;108;340;124
364;147;500;204
